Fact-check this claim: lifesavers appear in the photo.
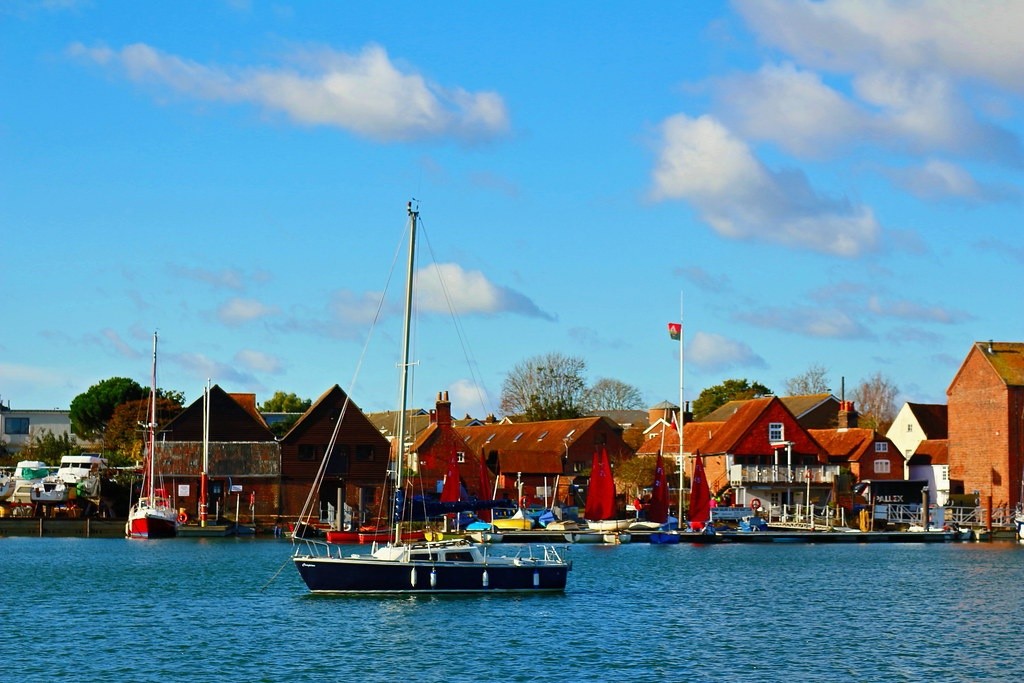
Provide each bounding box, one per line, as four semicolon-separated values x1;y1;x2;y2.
177;513;188;522
751;498;762;510
519;496;531;508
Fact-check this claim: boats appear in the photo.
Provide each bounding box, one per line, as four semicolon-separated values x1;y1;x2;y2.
0;447;109;518
469;532;503;543
562;531;603;543
602;530;631;544
323;516;639;546
658;515;678;531
650;530;681;545
907;525;991;541
1013;499;1024;542
629;521;661;531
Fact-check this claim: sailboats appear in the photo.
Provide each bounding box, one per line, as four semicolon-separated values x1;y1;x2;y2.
125;330;180;541
286;195;574;597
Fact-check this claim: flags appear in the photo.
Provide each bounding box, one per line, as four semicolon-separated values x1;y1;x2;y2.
668;322;681;341
